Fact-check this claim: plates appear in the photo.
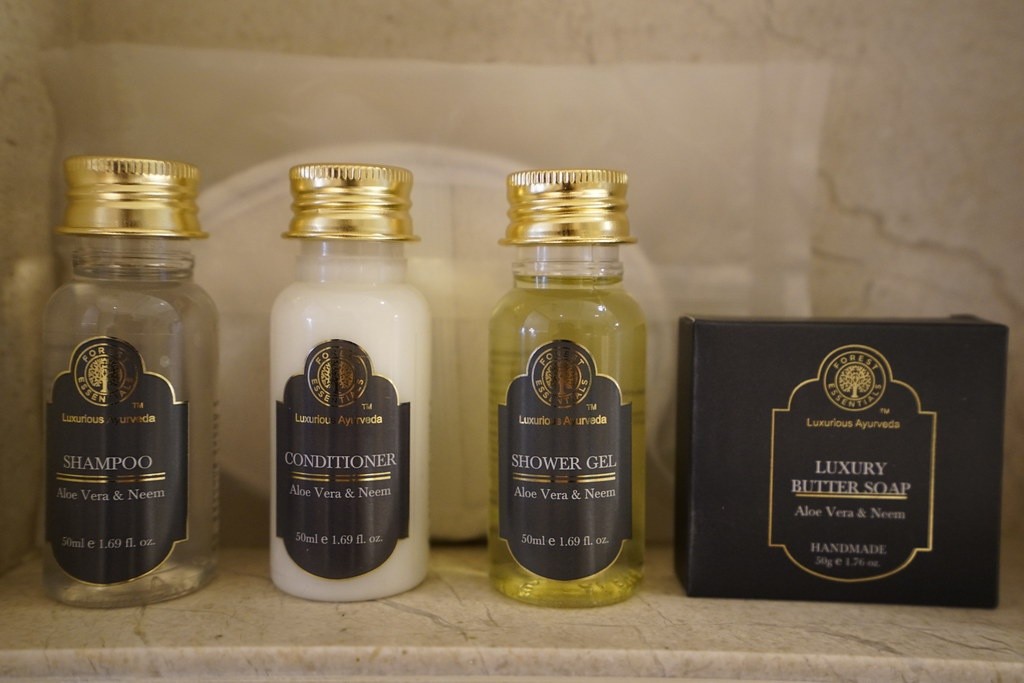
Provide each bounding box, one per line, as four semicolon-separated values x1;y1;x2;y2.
197;143;674;543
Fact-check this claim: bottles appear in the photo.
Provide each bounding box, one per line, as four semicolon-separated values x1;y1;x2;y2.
270;164;430;604
488;169;646;608
39;157;219;611
